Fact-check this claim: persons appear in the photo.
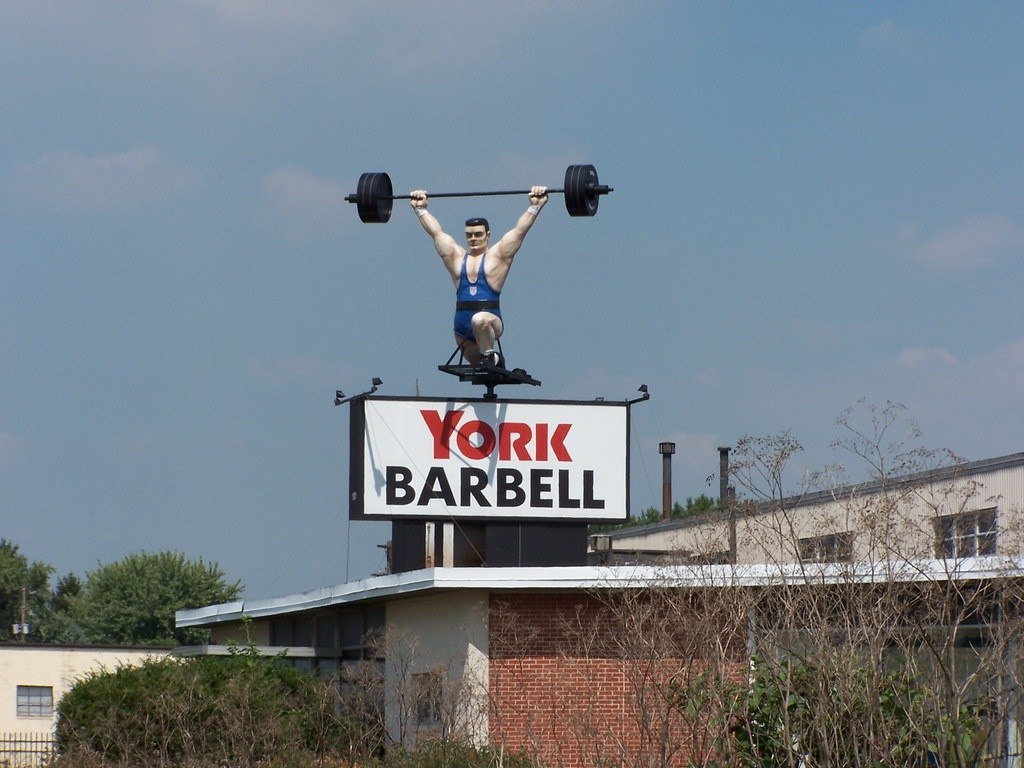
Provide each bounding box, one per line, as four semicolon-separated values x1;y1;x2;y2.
410;185;549;367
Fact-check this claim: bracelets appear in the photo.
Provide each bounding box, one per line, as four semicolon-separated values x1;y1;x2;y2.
415;207;427;218
527;204;541;216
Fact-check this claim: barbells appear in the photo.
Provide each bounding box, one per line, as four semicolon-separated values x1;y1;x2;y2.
344;164;614;224
